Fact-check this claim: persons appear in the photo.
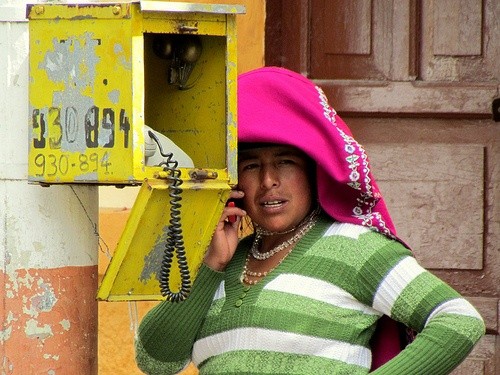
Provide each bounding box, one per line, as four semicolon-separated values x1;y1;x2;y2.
134;66;487;375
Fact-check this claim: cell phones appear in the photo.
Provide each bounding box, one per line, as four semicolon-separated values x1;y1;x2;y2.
226;198;241;222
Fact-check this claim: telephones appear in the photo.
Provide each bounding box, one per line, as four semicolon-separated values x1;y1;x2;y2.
145;125;194;167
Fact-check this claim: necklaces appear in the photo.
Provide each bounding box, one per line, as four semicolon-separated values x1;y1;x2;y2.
241;205;322;284
